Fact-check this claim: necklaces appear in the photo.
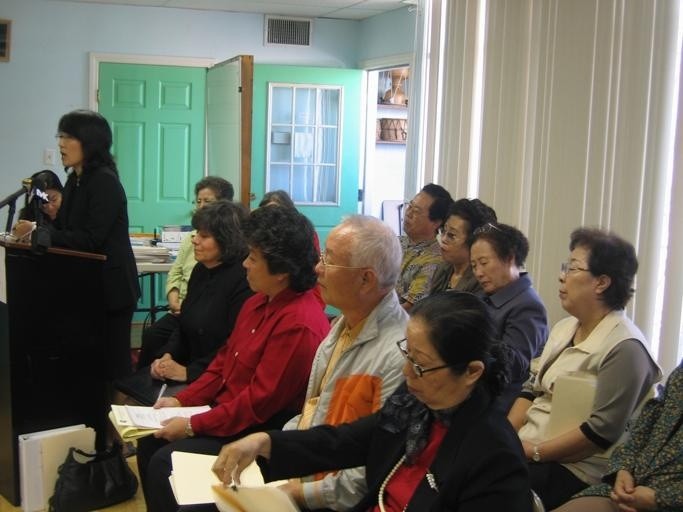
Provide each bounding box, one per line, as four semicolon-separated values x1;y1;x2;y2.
378;454;408;512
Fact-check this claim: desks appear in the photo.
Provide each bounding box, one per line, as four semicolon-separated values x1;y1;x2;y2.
123;231;196;374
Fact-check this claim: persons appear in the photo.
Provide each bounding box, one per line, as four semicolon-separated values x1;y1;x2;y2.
150;200;255;384
212;290;533;512
259;191;327;312
329;184;454;329
469;224;547;414
431;199;497;297
132;176;234;372
20;170;64;224
138;205;331;508
549;358;683;512
144;214;410;512
13;110;142;405
506;228;664;512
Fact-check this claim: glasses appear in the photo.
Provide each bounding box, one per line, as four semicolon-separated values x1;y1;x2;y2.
395;337;453;377
407;201;423;216
55;133;77;141
442;228;464;245
561;262;596;276
319;249;375;273
474;222;502;236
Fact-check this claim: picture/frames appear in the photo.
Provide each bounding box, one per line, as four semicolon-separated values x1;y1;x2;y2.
0;18;12;64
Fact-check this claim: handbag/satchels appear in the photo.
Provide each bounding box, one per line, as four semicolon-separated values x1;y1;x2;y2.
139;303;187;360
46;438;139;512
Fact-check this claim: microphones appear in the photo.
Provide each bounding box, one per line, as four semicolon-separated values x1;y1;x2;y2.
0;173;48;208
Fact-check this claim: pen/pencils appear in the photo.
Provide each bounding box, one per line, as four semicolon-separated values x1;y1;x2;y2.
230;480;238;491
156;383;168;402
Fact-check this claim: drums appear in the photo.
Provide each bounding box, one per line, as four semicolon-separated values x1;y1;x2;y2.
380;118;408;143
381;69;409;105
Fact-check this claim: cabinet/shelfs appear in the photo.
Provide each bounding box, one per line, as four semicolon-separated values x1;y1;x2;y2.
373;64;411;145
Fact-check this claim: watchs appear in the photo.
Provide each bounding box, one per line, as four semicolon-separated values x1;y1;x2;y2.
532;445;542;463
185;418;195;437
